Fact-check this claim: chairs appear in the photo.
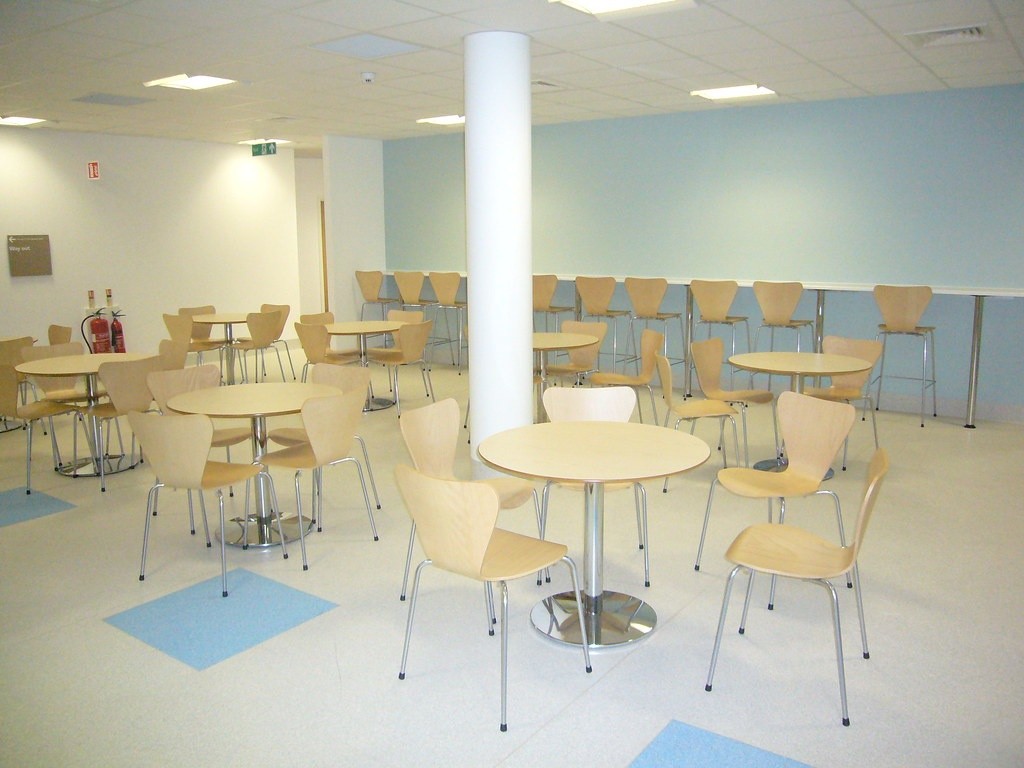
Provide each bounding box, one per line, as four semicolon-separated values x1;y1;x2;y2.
1;271;939;733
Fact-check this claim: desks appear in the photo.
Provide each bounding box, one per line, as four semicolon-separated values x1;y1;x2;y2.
166;383;341;550
321;321;403;413
534;332;599;423
723;351;872;483
193;312;250;387
478;423;710;646
13;353;154;479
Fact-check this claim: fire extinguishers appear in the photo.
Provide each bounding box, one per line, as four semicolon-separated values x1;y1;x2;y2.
111;310;126;353
81;308;112;354
